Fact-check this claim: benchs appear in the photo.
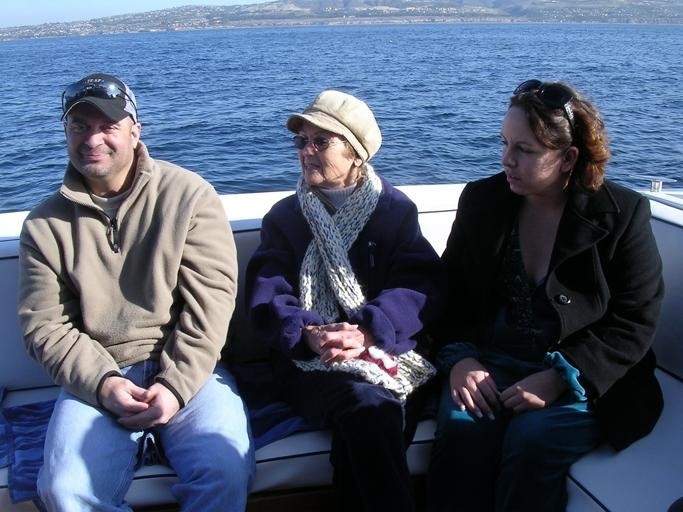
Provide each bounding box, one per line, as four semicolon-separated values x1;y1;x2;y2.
564;192;682;512
2;184;464;512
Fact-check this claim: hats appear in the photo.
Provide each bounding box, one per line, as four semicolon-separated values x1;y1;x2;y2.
286;90;382;163
61;74;138;124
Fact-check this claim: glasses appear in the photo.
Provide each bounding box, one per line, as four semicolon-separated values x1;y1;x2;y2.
63;80;137;117
513;80;575;134
291;135;346;152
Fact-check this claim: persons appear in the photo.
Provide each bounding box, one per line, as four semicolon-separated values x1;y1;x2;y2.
18;73;255;512
423;79;664;510
249;87;439;511
